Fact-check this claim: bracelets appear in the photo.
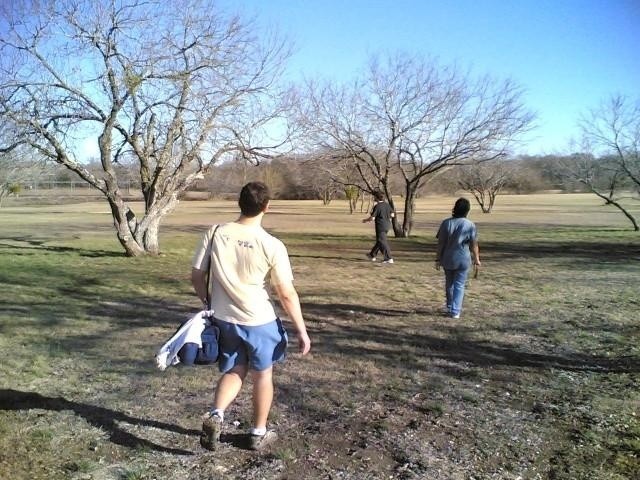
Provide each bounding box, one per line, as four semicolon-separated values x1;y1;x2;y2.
434;259;442;263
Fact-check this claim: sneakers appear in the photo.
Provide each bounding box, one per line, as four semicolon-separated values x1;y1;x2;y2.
447;305;461;319
366;252;394;264
248;430;278;451
199;415;222;452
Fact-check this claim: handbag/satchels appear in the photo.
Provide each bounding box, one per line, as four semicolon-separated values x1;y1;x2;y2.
173;313;221;366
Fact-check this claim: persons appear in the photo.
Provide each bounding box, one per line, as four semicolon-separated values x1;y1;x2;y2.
434;196;481;319
362;190;397;264
189;179;313;454
